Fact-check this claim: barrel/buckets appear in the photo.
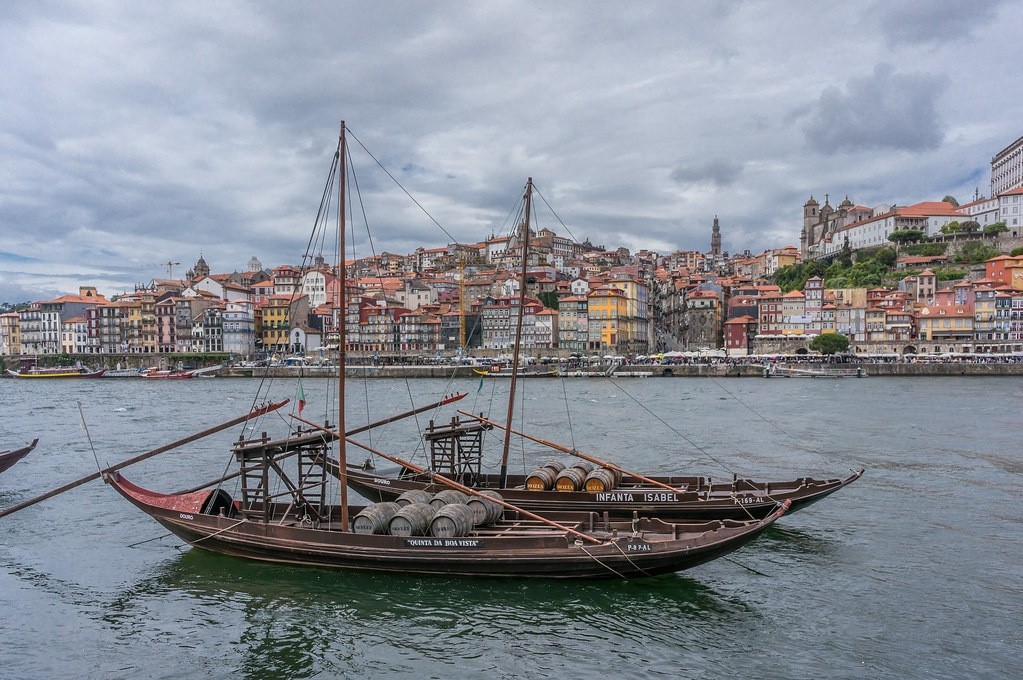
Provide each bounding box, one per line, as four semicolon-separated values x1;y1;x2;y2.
351;489;505;538
523;460;623;491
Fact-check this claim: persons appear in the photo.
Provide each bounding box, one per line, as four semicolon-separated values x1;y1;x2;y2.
563;356;1023;364
172;358;177;374
137;357;144;373
116;359;128;371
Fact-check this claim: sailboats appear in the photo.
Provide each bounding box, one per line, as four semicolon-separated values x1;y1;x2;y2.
307;177;866;518
76;119;791;583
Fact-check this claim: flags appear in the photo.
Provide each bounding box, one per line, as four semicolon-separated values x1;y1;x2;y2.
297;379;306;413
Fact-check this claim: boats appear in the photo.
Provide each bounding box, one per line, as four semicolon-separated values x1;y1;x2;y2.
140;362;195;379
472;368;558;379
3;349;106;379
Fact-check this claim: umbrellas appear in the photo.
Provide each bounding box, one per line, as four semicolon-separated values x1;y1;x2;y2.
528;349;723;361
